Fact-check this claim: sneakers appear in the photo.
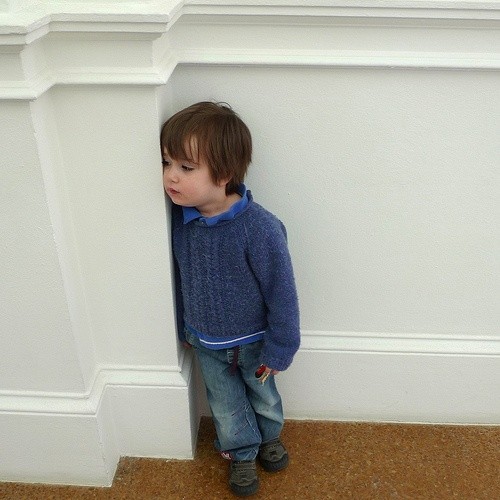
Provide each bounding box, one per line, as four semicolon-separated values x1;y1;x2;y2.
229;461;258;494
258;439;289;471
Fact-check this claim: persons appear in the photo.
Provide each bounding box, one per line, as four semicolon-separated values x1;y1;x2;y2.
160;101;302;497
